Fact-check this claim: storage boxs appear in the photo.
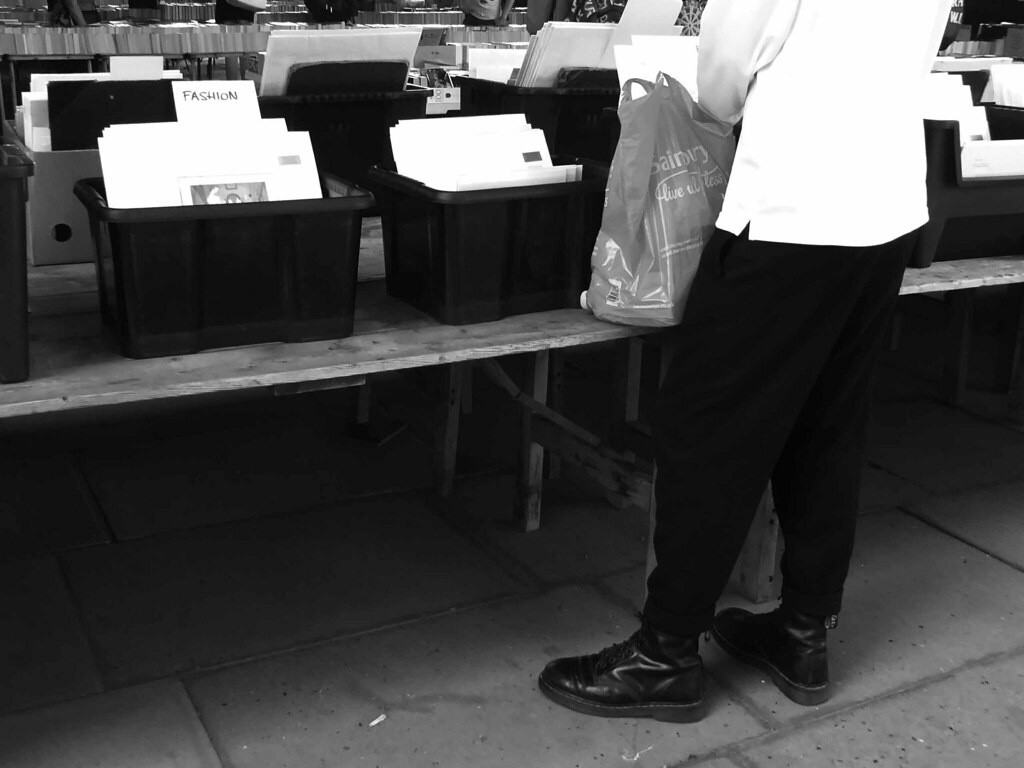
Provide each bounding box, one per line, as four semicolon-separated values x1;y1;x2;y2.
366;160;615;329
906;98;1023;268
71;173;379;359
5;121;107;269
0;124;31;383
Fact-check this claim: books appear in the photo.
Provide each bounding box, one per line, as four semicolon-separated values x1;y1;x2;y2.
944;37;1006;57
0;0;518;58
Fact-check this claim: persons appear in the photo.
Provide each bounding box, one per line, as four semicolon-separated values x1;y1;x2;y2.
458;0;518;27
537;0;960;724
48;0;110;72
214;0;255;79
525;0;627;166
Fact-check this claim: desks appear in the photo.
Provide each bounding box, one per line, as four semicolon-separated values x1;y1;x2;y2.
1;52;1023;602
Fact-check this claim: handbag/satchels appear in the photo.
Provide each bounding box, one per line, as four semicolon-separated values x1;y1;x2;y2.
590;72;736;325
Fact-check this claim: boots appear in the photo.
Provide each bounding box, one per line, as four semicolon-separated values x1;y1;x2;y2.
539;613;704;722
711;595;831;706
